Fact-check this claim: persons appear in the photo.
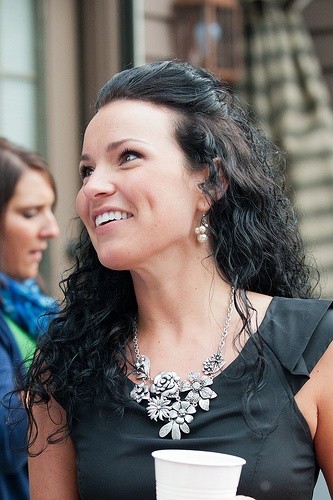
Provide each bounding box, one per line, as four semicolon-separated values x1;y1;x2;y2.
1;56;332;500
0;136;63;498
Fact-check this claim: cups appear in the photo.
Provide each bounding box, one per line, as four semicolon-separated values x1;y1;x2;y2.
151;449;246;500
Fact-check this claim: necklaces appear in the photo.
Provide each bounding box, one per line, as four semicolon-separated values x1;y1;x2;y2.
116;282;236;441
